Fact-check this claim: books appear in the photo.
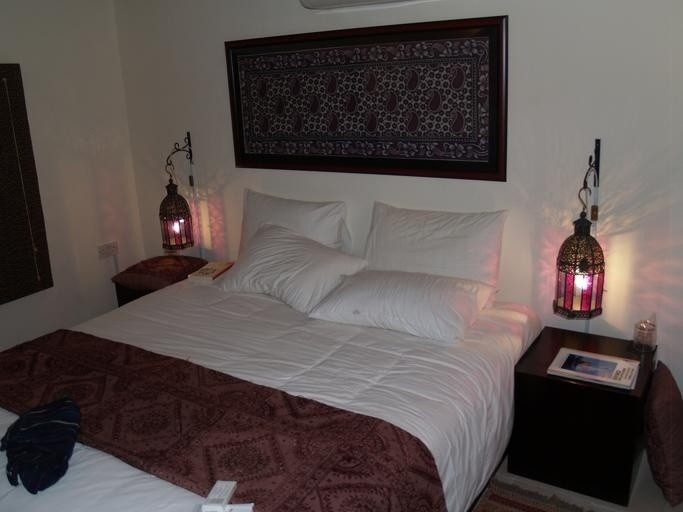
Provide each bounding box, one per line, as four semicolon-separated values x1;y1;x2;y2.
546;347;641;390
187;260;235;281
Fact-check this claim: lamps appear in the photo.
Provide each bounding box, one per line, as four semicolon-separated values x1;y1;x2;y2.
157;131;199;250
553;135;615;320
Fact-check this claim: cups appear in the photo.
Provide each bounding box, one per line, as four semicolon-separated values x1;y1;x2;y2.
633;321;658;353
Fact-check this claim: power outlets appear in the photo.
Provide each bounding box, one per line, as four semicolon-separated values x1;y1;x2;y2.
98;242;118;257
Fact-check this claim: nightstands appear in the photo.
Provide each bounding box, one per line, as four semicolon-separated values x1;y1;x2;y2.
508;326;658;485
112;255;208;307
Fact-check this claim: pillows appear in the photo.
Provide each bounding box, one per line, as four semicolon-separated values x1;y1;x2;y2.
368;202;509;308
240;188;346;272
221;224;365;312
306;269;497;343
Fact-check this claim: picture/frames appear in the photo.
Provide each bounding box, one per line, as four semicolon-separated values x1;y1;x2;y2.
222;15;507;183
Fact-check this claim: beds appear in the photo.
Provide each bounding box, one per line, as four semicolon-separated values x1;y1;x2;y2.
1;262;542;510
